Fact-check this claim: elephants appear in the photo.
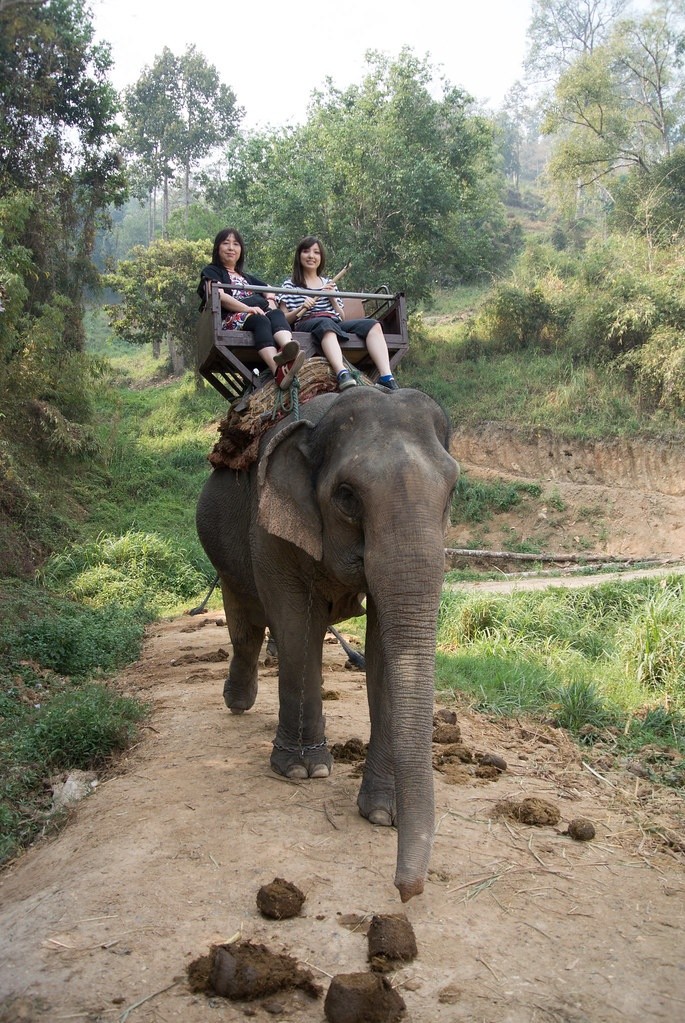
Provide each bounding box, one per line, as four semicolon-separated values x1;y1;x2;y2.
197;377;461;903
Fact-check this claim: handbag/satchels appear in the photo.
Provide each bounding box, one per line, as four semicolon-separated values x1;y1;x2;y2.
305;309;343;322
238;293;270;310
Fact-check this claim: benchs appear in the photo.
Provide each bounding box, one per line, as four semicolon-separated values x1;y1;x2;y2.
196;280;411;413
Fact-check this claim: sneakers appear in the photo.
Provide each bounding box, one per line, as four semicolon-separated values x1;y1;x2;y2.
273;340;300;367
338;372;356;390
374;377;400;393
274;349;306;390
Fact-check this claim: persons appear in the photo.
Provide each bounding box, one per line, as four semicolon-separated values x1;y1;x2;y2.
280;235;400;393
196;227;305;390
